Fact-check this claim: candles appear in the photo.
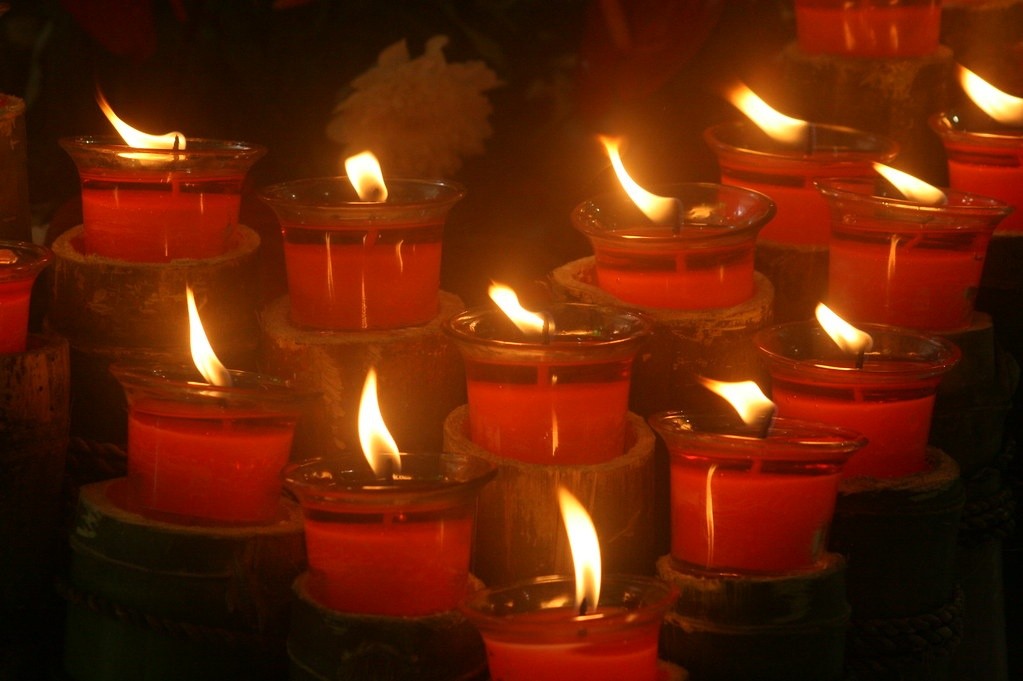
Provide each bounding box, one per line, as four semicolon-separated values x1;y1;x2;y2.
1;0;1019;681
